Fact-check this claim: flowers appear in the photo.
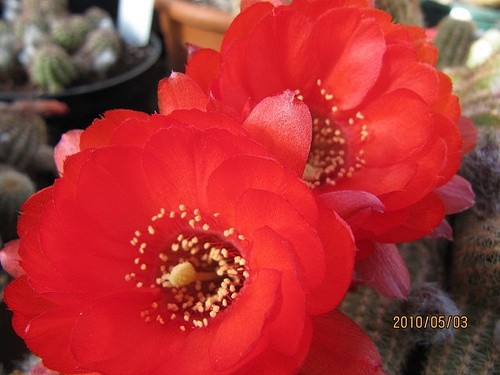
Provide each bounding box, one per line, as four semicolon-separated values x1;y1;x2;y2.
0;0;482;373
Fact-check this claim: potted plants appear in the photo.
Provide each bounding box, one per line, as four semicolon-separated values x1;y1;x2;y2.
0;0;168;149
0;104;60;375
155;0;240;73
